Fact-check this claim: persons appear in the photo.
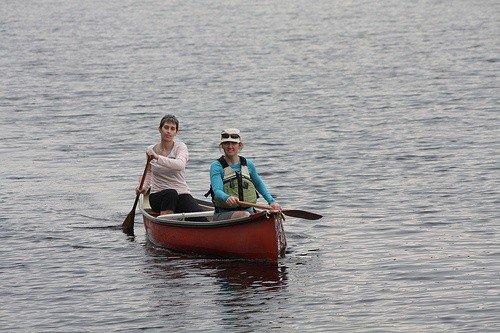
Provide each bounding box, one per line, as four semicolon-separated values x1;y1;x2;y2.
136;115;209;222
209;128;283;221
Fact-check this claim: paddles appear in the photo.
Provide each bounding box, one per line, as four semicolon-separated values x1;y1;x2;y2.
237;199;325;220
121;155;151;228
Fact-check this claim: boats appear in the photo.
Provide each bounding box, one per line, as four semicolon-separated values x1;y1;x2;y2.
139;175;287;260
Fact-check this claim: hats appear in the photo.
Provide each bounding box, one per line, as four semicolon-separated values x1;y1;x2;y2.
219;128;240;144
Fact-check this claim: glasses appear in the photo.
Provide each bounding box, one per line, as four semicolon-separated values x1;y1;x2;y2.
221;133;240;139
